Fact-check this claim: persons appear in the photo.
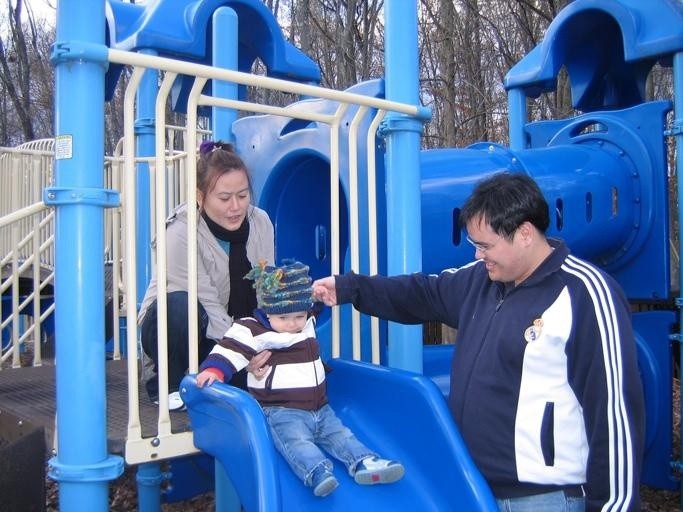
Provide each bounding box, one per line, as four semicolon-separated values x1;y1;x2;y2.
310;172;646;512
195;260;405;496
137;139;275;409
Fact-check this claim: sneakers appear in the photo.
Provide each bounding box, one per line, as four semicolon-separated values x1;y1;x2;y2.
311;462;340;497
152;391;187;412
354;456;405;485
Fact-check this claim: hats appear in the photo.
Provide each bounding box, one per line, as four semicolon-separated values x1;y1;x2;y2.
242;258;313;314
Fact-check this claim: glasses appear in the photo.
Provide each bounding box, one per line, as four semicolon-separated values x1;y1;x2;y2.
466;234;507;255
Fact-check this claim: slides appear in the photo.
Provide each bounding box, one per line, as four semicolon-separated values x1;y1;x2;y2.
179;357;499;511
